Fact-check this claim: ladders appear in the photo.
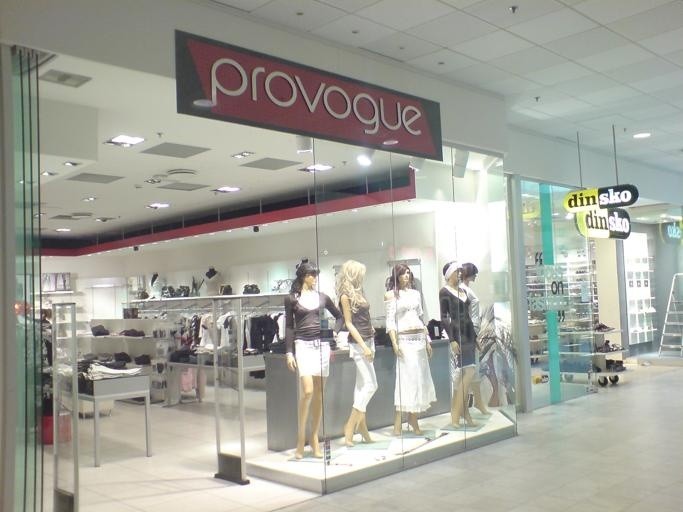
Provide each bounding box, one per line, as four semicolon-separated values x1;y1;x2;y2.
657;273;683;358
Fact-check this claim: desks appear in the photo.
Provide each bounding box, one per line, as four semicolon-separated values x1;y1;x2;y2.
35;374;152;468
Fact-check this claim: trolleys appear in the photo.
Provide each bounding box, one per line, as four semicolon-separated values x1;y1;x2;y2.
539;323;625;389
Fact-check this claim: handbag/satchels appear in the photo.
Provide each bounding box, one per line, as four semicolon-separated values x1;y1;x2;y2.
272;278;294;295
40;272;71;292
242;283;260;294
137;291;149;299
174;285;189;297
160;285;175;298
218;284;232;295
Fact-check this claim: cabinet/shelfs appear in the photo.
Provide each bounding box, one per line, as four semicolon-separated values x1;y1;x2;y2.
525;262;629;387
30;290;292;407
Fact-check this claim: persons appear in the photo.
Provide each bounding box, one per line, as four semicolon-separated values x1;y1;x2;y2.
148;271;163;298
448;262;493;416
334;258;380;447
438;260;483;429
284;261;345;459
383;263;437;436
203;266;222;295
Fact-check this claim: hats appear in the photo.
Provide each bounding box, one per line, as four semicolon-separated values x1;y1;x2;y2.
441;260;463;282
295;261;320;277
